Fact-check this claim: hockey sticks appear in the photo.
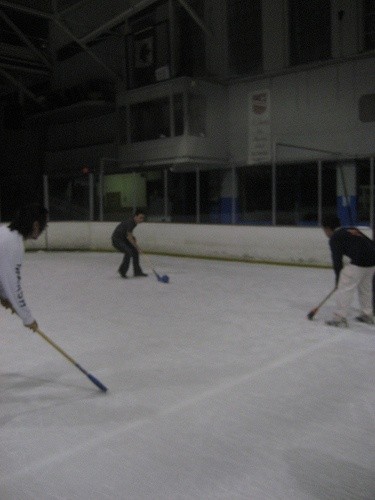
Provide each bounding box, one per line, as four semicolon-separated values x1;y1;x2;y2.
135;244;162;281
6;299;109;394
307;288;338;320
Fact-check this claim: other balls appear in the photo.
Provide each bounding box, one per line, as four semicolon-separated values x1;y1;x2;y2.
161;275;169;282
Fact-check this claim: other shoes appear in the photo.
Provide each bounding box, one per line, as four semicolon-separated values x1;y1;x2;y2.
355;316;374;323
327;318;348;328
117;268;129;279
133;272;147;277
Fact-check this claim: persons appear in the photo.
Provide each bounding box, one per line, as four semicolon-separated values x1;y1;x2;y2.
0;199;48;334
320;213;375;328
111;209;149;278
